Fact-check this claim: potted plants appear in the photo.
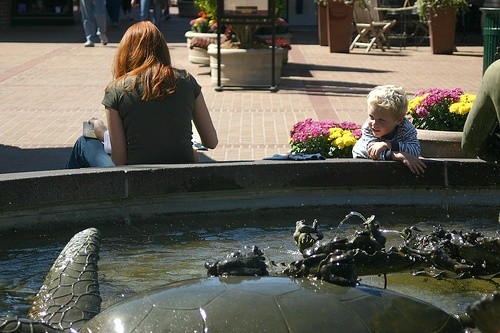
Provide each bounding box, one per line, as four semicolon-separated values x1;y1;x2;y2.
312;0;473;55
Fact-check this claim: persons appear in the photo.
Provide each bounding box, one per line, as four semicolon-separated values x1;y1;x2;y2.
18;0;170;23
130;0;162;33
63;19;218;170
350;85;429;176
78;0;108;47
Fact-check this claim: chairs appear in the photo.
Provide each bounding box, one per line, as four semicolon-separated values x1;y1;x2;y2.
349;0;397;53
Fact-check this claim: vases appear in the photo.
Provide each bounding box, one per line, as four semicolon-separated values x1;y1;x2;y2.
185;31;291;65
414;128;478;160
206;43;284;87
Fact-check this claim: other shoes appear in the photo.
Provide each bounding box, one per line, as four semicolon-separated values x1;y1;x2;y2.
85;34;95;47
99;34;109;45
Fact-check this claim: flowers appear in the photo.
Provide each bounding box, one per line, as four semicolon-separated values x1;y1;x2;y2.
406;87;478;132
189;0;289;46
288;117;362;159
